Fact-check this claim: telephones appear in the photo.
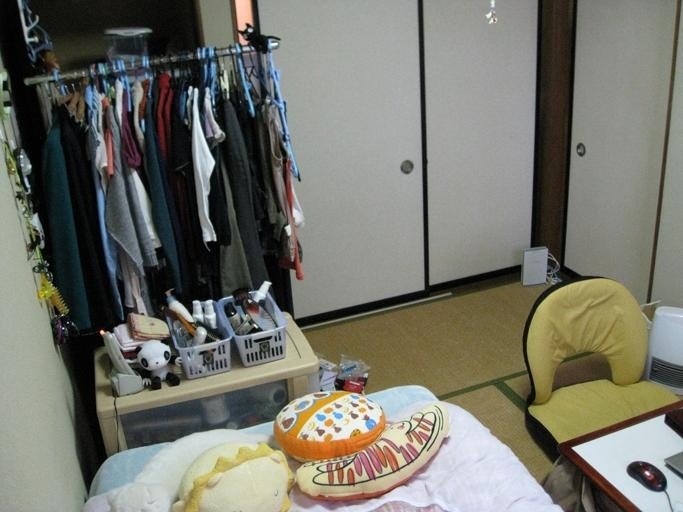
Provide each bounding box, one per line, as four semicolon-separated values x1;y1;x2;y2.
103;331;144;397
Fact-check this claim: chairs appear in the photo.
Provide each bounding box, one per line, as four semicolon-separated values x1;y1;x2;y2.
517;265;680;457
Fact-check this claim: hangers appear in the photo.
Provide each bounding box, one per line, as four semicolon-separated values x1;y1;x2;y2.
26;18;305;185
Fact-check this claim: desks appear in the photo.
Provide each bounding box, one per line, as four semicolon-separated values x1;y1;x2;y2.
557;393;683;512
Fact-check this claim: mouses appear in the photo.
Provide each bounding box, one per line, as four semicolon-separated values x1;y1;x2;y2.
628;461;667;492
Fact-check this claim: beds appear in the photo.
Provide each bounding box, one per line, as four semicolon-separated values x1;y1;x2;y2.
72;378;564;512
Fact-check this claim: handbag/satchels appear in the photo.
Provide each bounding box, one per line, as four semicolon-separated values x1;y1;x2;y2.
521;246;560;286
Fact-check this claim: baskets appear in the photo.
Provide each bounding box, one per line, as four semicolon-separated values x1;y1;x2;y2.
218;291;287;367
164;300;232;379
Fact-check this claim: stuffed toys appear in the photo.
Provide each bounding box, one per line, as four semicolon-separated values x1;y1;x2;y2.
132;336;183;390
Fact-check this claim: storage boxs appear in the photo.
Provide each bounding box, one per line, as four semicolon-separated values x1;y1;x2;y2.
82;311;324;464
